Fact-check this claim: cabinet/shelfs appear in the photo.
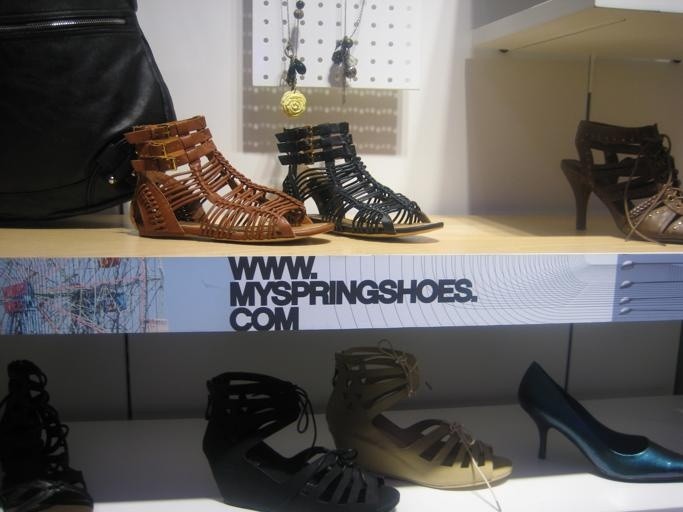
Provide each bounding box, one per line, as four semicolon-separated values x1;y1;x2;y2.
3;2;683;507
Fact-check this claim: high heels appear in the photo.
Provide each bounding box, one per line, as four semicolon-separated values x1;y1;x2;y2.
560;119;683;246
518;361;683;482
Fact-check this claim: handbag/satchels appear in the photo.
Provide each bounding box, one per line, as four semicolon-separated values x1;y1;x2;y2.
0;1;178;231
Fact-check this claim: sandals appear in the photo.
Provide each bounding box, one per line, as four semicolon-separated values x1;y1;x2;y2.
0;360;93;511
326;344;514;488
275;121;443;240
124;115;336;243
202;371;400;512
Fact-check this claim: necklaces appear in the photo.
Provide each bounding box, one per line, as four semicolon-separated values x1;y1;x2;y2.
327;1;364;109
279;0;308;119
